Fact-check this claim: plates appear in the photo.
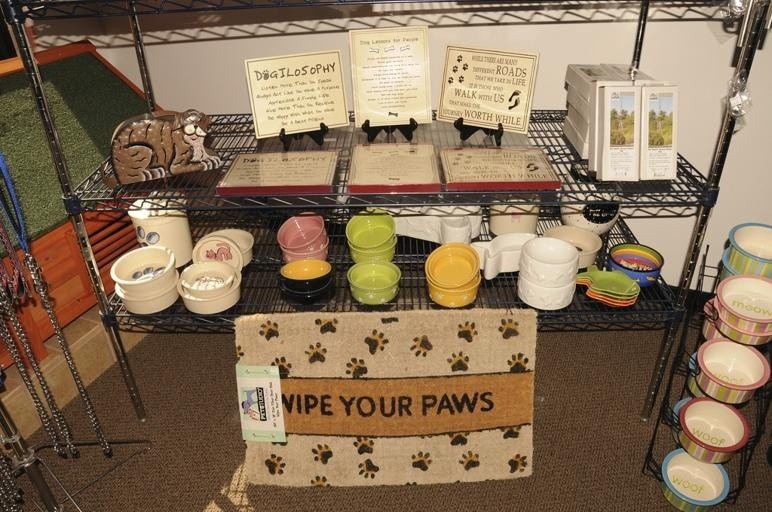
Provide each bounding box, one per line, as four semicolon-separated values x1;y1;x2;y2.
580;268;639;308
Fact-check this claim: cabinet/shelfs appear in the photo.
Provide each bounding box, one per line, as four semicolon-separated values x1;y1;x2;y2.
2;0;771;426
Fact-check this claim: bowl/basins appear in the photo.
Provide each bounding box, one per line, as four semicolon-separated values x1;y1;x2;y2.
515;237;578;310
104;208;401;315
659;224;772;511
421;242;481;308
608;243;664;288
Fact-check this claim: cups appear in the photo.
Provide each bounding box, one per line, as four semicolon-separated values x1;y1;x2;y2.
443;215;468;244
